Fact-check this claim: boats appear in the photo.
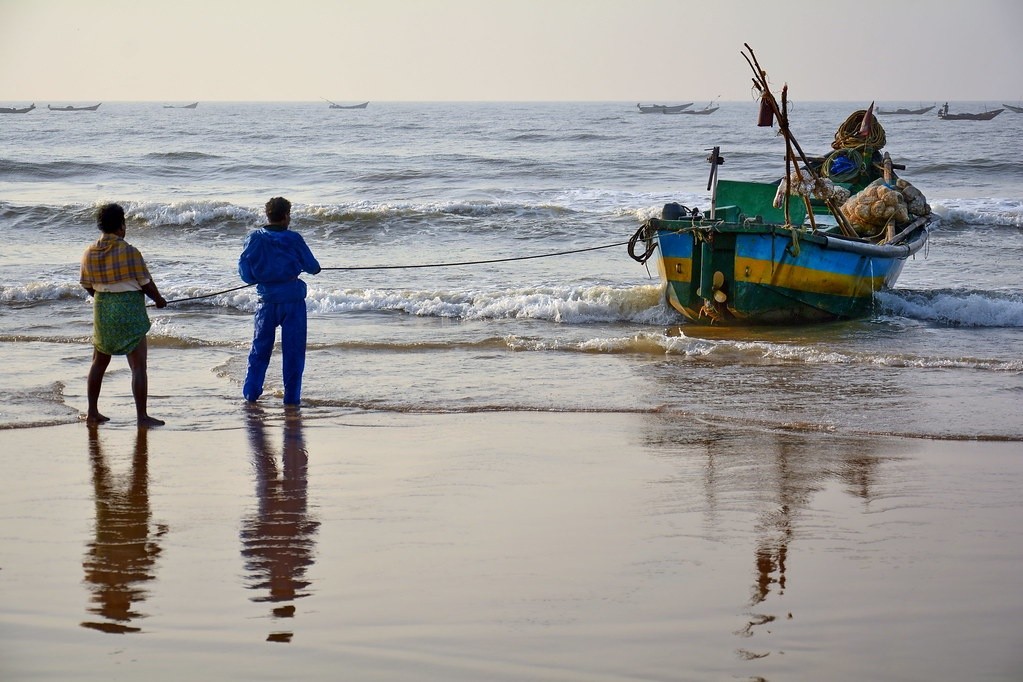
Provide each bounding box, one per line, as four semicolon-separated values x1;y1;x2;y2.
47;102;102;111
938;108;1005;120
164;101;198;108
875;106;935;114
1002;103;1023;113
636;103;693;113
662;95;722;115
320;96;369;109
0;102;36;114
641;41;936;324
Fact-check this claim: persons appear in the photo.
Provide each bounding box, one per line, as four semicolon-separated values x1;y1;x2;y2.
239;197;322;405
79;203;167;425
943;102;948;115
